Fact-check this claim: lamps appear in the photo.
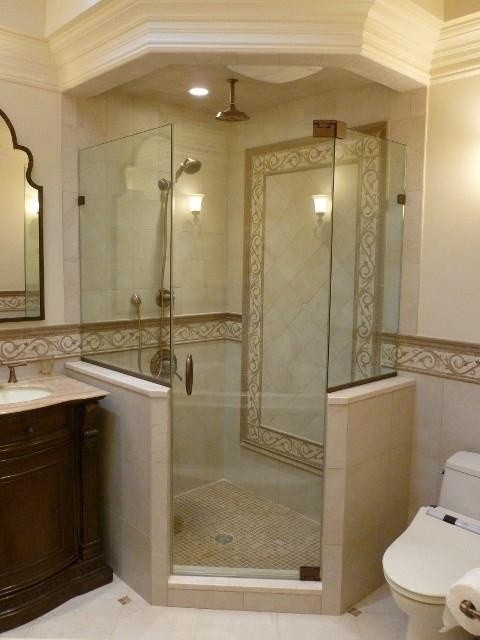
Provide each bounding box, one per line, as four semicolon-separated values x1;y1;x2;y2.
186;188;206;224
309;191;332;229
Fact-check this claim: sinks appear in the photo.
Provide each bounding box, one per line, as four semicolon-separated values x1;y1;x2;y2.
0;385;54;404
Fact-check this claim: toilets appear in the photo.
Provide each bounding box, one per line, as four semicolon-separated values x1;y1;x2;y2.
382;451;480;640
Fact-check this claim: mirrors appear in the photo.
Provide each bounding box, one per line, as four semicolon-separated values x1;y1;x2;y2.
256;161;359;448
0;107;47;323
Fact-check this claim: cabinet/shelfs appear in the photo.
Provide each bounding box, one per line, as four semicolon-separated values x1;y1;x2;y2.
3;399;77;637
